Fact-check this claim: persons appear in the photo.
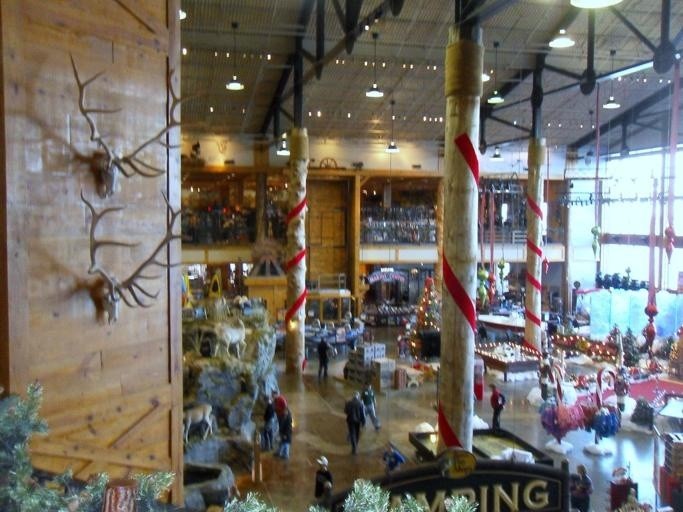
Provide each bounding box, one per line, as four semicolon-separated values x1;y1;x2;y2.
360;381;381;431
271;390;289;426
313;455;333;504
259;396;278;452
316;337;332;381
343;390;366;455
569;464;594;512
488;382;506;429
272;408;293;461
381;446;406;477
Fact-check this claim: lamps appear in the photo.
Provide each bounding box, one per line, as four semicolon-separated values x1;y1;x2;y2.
385;100;399;153
365;32;384;98
225;22;244;91
602;50;621;109
487;41;504;105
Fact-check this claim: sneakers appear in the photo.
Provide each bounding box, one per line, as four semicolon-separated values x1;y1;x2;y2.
272;452;290;460
350;445;360;457
375;425;382;430
261;446;274;452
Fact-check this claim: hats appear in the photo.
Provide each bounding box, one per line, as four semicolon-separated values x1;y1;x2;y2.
316;455;329;466
